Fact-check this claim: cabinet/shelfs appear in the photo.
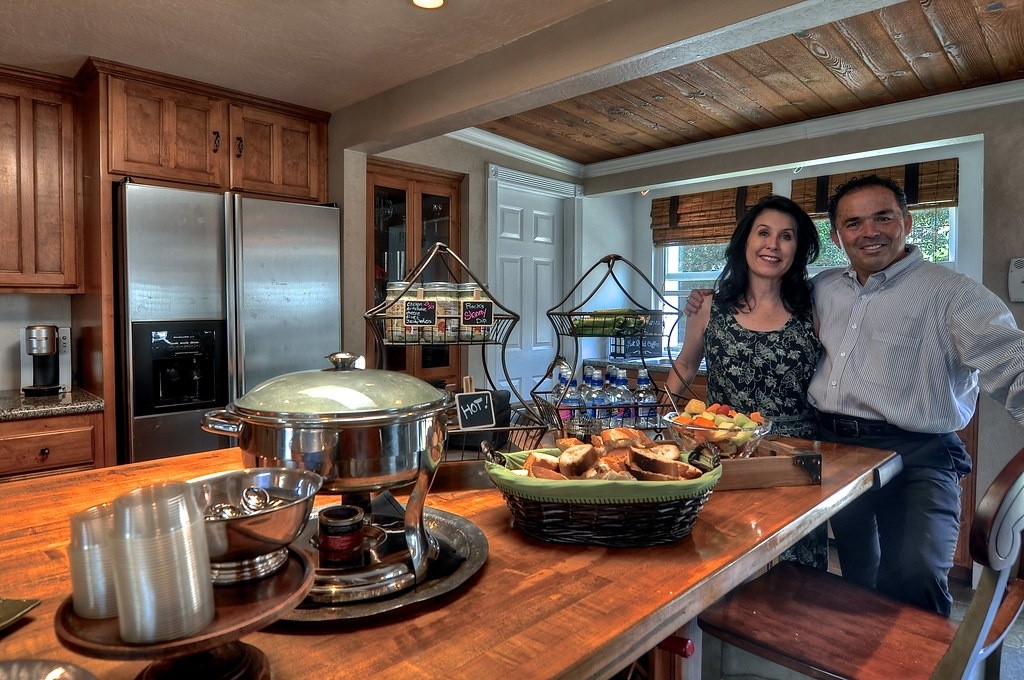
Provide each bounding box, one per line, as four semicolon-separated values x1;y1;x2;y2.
582;366;711;418
0;410;105;484
73;56;333;206
0;62;86;295
365;153;463;394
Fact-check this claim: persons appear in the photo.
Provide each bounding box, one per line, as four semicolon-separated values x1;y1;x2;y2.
683;173;1024;617
659;194;830;574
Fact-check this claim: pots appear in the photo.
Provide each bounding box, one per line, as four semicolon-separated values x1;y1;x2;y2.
199;350;459;493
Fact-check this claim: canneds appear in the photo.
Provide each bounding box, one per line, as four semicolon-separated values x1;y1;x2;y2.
385;281;494;342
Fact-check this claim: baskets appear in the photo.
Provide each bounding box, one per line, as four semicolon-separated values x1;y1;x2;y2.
481;440;722;544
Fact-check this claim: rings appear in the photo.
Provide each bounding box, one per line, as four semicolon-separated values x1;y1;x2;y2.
686;296;691;302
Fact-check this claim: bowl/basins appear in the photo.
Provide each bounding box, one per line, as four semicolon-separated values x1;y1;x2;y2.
175;466;323;584
68;483;219;644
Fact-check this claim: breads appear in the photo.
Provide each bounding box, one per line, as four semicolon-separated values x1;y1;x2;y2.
520;426;703;482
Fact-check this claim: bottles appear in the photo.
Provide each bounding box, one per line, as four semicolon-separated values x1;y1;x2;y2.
386;280;424;342
553;363;657;432
421;281;461;345
457;281;494;341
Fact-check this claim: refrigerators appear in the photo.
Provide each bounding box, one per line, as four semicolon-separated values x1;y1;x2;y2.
113;175;344;464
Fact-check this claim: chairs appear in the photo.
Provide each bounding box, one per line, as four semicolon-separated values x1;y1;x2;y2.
694;450;1024;680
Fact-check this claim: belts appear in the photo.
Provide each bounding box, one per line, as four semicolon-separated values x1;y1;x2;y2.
816;409;902;437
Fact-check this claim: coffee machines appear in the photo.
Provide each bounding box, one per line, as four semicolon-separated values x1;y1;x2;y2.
17;324;73;398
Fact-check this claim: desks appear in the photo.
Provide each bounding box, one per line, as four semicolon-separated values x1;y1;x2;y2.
0;428;904;680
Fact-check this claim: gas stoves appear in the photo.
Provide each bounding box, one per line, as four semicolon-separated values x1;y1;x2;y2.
258;446;492;625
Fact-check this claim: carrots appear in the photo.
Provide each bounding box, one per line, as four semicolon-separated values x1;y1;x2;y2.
673;416;714;436
